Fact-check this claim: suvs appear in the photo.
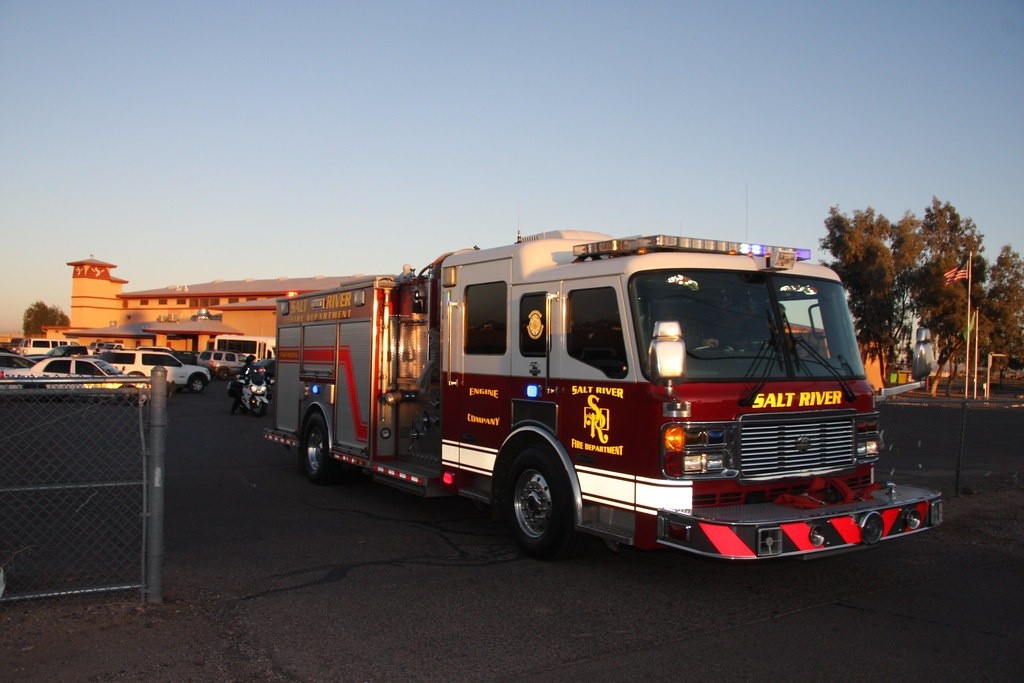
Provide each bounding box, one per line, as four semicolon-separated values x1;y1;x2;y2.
99;350;212;393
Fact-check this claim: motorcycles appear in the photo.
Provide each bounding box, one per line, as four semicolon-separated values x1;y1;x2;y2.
227;363;270;416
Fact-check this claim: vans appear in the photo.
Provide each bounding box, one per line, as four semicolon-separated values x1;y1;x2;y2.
16;338;81;357
137;346;175;355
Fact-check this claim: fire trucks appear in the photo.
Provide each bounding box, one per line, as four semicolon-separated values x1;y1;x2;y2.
258;228;946;565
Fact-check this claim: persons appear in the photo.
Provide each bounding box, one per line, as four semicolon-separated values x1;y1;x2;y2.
588;318;627;363
228;354;257;415
682;335;720;349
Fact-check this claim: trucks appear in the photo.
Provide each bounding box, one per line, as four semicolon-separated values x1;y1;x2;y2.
215;335;275;360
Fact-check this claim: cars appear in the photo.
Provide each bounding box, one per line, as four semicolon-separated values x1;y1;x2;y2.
254;360;275;384
0;342;124;364
0;356;152;407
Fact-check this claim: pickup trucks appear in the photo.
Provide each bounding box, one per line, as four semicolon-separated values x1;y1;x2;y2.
197;350;247;379
26;345;100;361
